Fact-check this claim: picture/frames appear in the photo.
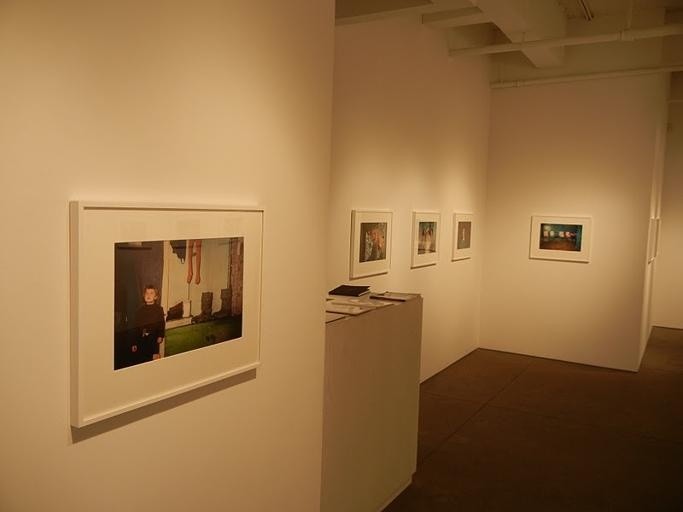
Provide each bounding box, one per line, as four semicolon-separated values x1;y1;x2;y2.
351;209;392;279
528;213;591;264
412;210;473;269
66;196;265;431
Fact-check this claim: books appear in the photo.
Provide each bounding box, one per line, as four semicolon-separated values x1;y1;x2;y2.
326;285;419;316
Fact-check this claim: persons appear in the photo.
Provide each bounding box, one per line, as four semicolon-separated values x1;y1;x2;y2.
131;286;164;366
422;224;433;253
187;239;202;284
366;228;385;261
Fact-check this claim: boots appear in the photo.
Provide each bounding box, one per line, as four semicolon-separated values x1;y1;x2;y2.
213;288;233;318
191;291;215;324
